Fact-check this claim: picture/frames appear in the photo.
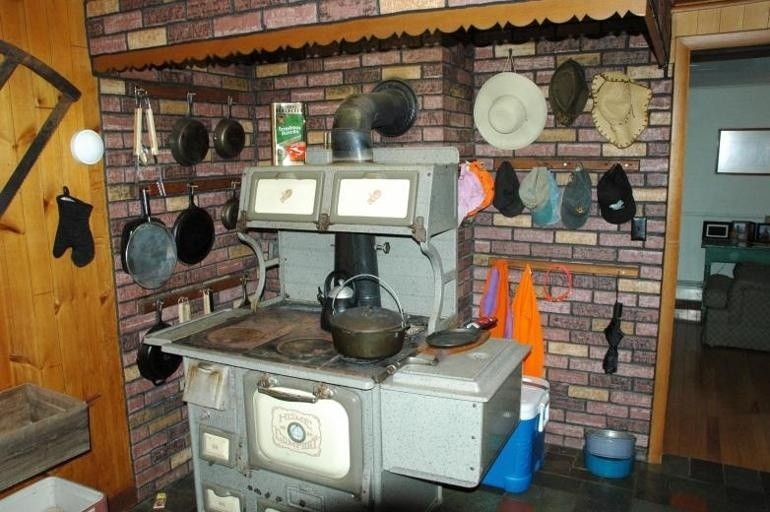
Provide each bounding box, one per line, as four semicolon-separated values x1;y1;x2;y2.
703;220;770;242
715;127;770;176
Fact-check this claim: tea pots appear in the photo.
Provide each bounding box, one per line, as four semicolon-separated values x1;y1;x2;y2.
316;269;358;331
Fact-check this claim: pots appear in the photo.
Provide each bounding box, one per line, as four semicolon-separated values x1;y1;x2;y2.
212;98;247;159
169;92;209;166
120;188;182;291
329;274;409;358
177;185;214;264
222;180;240;232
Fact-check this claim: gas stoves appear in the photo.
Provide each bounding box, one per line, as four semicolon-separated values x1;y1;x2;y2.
170;297;449;380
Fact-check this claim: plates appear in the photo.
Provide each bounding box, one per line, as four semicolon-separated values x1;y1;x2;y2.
426;328;479;348
585;428;636;459
581;443;635;479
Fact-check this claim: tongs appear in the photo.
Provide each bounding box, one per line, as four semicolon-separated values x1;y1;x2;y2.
129;87;162;181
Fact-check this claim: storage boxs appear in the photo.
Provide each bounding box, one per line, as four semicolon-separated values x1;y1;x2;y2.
481;375;551;494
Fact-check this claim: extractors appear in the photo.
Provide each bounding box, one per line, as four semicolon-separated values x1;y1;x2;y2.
235;81;463;236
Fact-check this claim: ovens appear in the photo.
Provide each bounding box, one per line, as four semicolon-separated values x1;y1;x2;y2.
182;359;381;512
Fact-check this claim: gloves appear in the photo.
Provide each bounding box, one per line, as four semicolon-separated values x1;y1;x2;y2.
53;195;95;266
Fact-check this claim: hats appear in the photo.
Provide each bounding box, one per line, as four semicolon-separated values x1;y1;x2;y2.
518;166;560;226
493;161;524;218
472;72;548;151
458;161;495;225
560;164;592;228
597;164;635;224
591;72;652;147
549;57;587;124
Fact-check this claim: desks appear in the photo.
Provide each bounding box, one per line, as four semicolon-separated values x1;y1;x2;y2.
700;243;770;327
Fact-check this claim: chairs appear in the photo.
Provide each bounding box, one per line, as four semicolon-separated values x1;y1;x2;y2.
700;262;769;353
